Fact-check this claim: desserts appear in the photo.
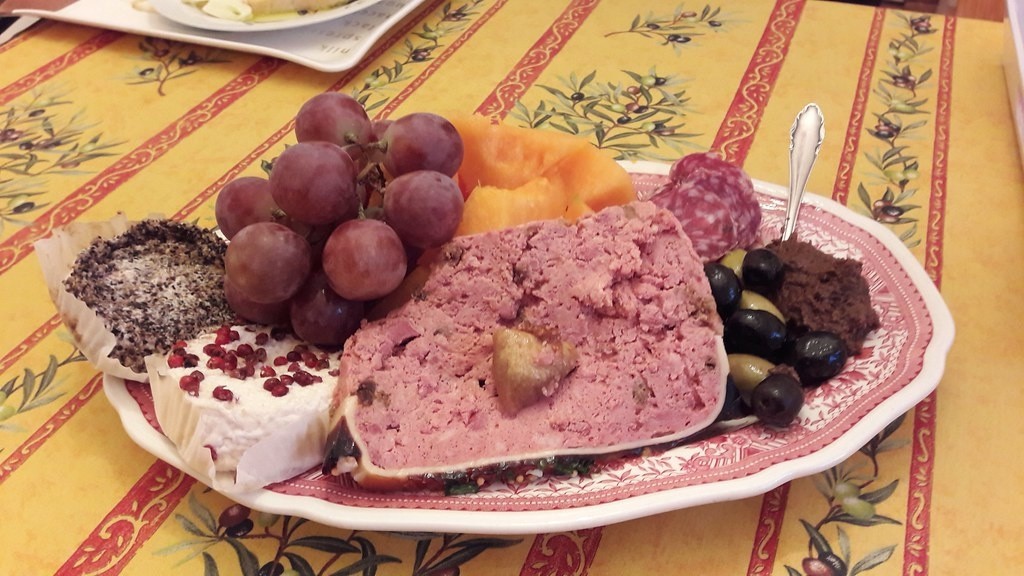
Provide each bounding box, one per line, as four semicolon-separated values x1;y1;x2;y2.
144;315;343;498
32;209;245;383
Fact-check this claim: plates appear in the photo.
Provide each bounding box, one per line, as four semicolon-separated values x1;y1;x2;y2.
145;0;381;30
101;162;953;535
13;0;424;73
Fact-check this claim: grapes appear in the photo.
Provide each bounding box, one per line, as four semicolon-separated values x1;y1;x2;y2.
214;89;466;346
